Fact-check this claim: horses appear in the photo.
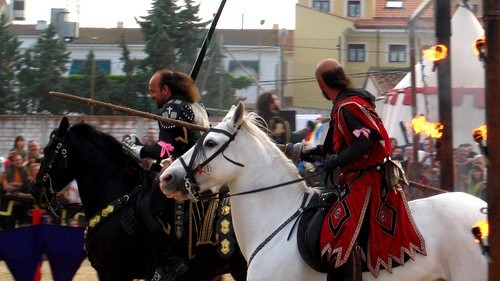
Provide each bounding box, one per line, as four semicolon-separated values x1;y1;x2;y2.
160;103;489;281
32;117;248;281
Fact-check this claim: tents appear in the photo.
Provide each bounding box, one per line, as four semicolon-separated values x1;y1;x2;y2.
381;2;488;166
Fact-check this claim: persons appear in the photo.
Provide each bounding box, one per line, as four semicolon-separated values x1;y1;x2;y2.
255;90;490;203
122;70;223;280
275;56;391;280
1;125;162;230
